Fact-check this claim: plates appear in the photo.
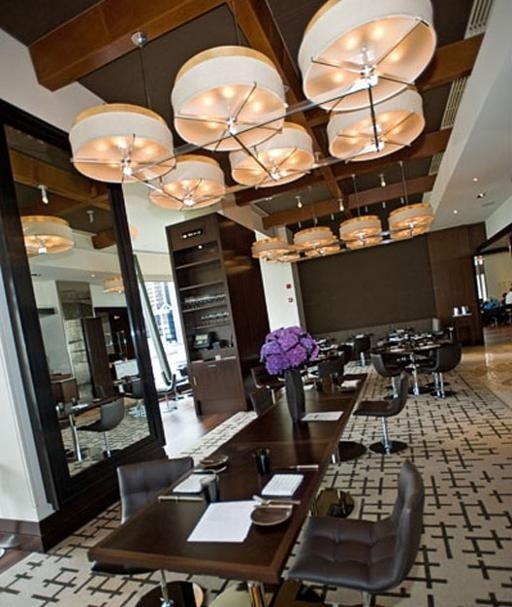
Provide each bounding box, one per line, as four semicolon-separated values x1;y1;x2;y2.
200;454;229;467
249;503;293;527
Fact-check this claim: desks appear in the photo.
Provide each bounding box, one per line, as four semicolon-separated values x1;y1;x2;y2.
91;442;354;606
224;373;367;463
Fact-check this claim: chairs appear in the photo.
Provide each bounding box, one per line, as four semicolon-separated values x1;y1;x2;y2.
250;386;275;418
91;457;205;606
286;459;423;606
78;395;124;460
114;371;194;410
314;325;463;400
352;372;409;454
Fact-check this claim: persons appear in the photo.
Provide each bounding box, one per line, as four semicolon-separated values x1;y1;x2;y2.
481;298;494;324
505;288;512;305
501;293;508;306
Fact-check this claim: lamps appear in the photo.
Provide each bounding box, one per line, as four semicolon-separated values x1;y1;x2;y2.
252;160;434;263
21;214;76;258
69;0;441;212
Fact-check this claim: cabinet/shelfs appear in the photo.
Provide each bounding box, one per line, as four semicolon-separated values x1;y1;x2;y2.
165;212;271;417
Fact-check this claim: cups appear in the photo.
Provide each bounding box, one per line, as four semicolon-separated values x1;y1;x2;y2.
252;448;272;474
198;475;219;504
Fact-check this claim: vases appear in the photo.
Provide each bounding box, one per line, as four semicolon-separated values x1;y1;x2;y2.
284;373;306;422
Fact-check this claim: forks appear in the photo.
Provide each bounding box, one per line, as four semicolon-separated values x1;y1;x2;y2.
190;466;227;474
253;495;301;505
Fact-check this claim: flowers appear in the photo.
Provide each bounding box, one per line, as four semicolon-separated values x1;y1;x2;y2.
258;325;320;376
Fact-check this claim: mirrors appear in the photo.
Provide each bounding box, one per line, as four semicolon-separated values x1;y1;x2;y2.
0;97;168;513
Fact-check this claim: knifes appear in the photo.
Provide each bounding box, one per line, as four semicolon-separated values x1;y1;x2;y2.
272;464;319;468
158;495;204;501
